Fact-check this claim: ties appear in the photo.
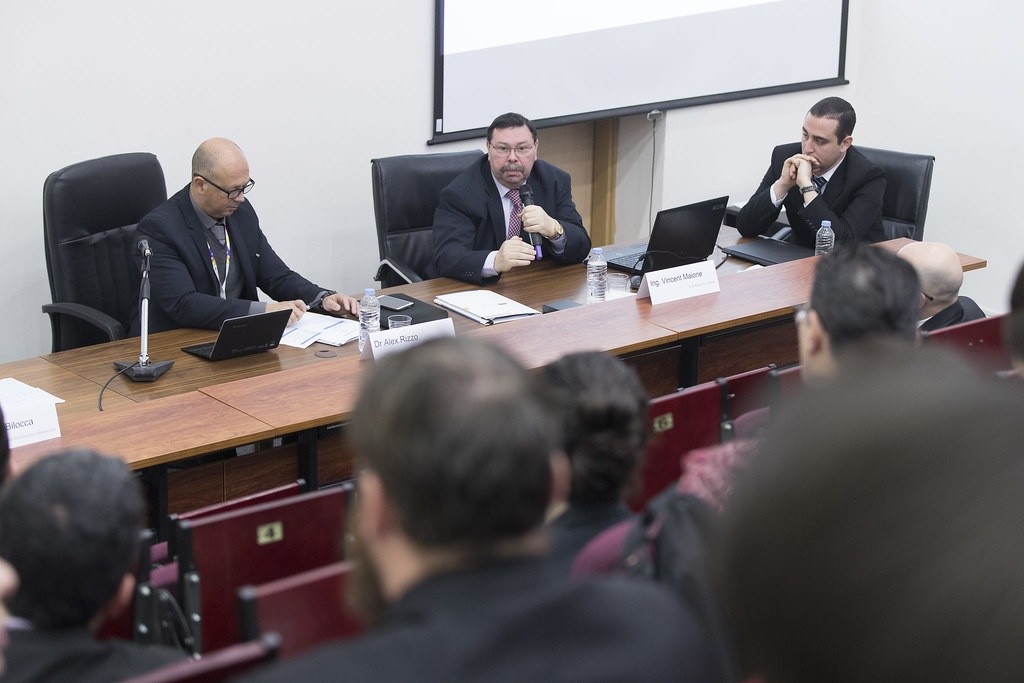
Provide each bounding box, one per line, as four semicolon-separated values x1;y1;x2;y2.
812;175;827;191
506;189;523;239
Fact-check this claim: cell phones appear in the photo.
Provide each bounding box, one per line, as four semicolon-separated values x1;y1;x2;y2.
376;295;414;311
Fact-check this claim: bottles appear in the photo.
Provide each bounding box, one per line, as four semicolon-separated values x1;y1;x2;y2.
358;288;380;353
587;248;607;305
815;220;835;256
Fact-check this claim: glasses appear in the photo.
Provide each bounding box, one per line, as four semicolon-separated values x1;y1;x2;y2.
194;173;255;199
489;142;535;154
793;304;811;329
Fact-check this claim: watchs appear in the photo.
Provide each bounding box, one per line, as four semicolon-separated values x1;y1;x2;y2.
545;222;563;240
800;182;819;194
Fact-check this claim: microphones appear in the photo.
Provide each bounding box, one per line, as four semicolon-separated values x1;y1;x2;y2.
519;184;542;260
133;234;151;256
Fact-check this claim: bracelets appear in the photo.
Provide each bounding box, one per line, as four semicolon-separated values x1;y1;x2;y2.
320;290;337;309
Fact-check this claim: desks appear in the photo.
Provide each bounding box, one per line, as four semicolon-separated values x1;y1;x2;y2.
1;227;984;526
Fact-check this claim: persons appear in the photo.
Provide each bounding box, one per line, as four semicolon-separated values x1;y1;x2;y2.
896;241;987;332
539;352;649;567
427;113;592;286
735;97;886;251
129;138;360;339
0;447;187;683
253;335;699;683
795;244;920;385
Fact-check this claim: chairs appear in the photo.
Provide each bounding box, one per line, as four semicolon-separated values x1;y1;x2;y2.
97;312;1024;683
42;152;168;353
371;148;484;289
771;144;936;245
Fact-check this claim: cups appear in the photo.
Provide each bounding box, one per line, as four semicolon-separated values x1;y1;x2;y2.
388;315;412;330
607;273;629;300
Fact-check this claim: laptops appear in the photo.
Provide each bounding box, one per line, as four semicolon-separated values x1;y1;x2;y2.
584;195;729;276
181;308;293;361
722;238;815;266
